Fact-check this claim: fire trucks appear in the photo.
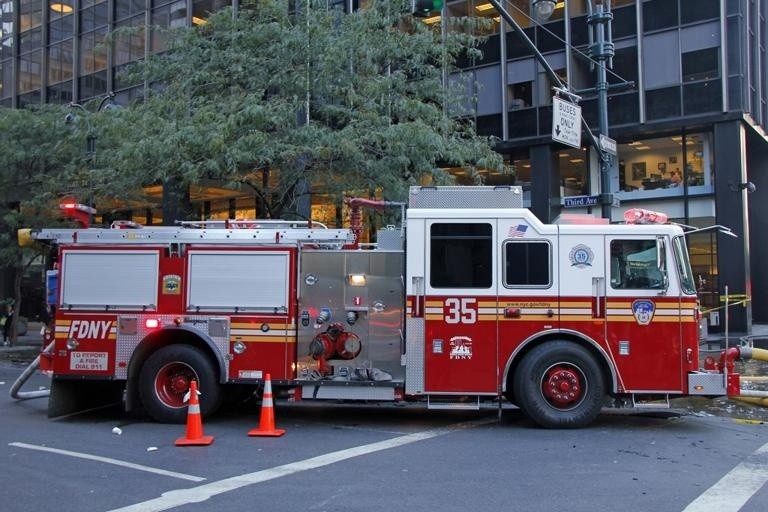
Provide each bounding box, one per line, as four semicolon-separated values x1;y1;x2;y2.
14;183;740;430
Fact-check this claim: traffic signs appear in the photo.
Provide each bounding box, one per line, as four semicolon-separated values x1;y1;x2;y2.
596;134;616;157
551;95;583;151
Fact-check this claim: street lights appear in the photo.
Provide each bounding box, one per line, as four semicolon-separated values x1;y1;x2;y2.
531;0;637;228
63;92;118;228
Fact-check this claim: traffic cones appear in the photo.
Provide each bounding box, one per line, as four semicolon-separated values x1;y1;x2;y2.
173;379;216;448
244;372;286;439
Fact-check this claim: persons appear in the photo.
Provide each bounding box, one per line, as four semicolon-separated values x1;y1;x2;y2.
669;170;681;184
3;304;15;346
675;166;681;178
